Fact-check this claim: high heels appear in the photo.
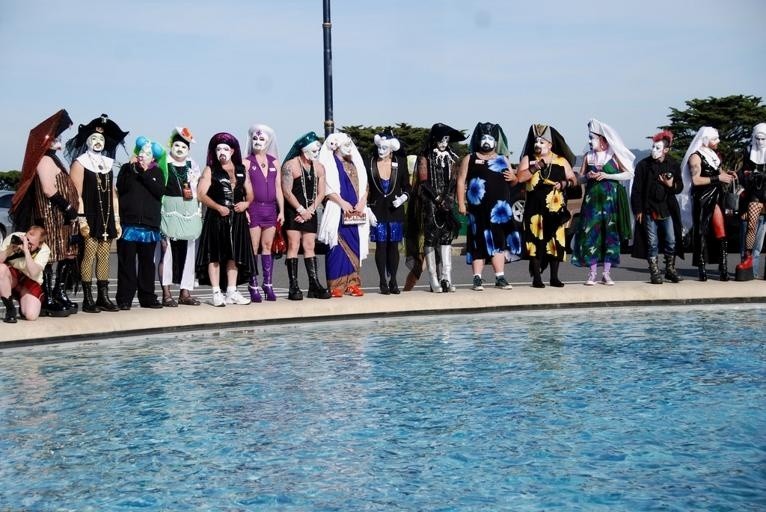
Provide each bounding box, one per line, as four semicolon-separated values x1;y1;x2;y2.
735;256;753;281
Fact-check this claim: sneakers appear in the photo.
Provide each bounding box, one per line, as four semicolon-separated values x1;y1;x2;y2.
213;291;226;306
226;290;251;305
345;283;363;296
471;258;613;291
328;287;343;297
119;291;200;310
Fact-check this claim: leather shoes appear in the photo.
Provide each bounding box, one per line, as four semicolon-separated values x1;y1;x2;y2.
379;281;389;294
388;281;401;294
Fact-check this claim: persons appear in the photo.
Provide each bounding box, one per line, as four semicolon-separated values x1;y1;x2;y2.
364;119;636;295
631;123;766;284
1;110;370;323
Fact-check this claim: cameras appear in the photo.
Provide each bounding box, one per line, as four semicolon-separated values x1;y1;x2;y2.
665;172;673;179
11;234;23;245
136;156;143;162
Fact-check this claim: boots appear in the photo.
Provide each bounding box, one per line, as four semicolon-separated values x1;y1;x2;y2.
285;258;303;300
718;242;729;280
261;255;277;301
40;268;121;316
698;246;708;280
438;245;456;292
663;253;684;283
248;255;263;303
646;256;663;284
423;247;443;292
304;256;332;299
1;294;17;323
